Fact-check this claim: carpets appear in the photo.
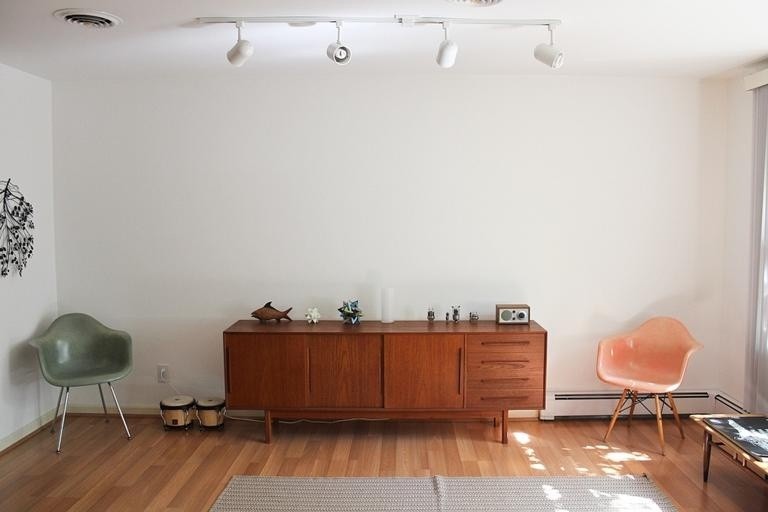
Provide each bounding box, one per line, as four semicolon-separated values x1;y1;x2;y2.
207;473;687;512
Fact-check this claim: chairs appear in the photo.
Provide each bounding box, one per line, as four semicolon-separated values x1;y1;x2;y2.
27;313;136;454
595;316;704;454
222;318;548;442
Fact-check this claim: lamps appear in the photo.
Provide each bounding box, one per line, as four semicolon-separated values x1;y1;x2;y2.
194;18;568;71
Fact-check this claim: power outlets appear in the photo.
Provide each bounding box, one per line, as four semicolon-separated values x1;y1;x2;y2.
155;363;170;382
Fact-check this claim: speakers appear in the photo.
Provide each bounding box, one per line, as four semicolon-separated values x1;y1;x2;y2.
496;304;530;325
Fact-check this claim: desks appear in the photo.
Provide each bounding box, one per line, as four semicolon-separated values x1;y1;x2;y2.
689;414;767;498
